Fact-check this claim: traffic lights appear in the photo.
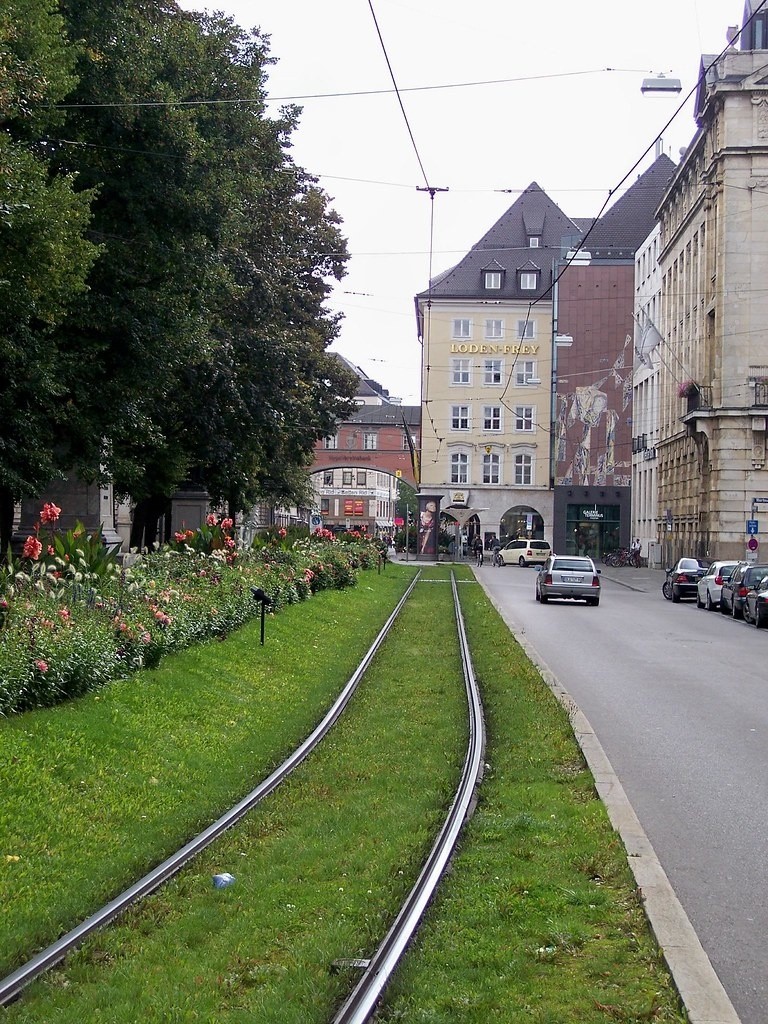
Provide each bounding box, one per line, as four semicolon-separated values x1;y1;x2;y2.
408;511;413;524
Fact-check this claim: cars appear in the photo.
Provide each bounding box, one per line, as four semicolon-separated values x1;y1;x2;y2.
566;540;579;556
496;539;552;567
742;574;768;628
665;557;731;603
696;561;742;611
731;565;768;619
720;563;768;615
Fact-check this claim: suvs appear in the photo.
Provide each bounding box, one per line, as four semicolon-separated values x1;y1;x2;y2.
535;553;601;606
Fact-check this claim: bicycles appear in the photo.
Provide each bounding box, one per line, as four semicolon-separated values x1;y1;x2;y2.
471;548;483;567
601;547;644;567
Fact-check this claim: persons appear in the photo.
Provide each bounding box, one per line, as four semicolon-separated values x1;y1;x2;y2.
631;539;642;568
419;501;436;554
383;535;395;549
475;535;483;565
490;535;501;567
